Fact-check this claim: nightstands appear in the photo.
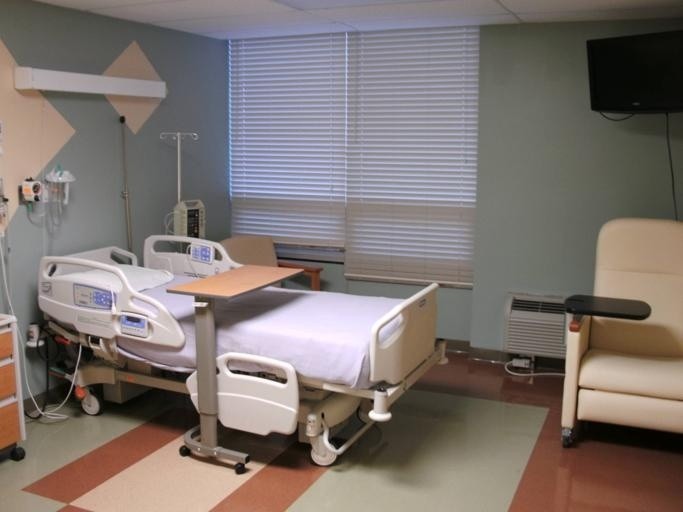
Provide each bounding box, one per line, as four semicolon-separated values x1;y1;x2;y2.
0;313;26;461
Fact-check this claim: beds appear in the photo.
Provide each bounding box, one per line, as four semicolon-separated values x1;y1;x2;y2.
38;234;449;477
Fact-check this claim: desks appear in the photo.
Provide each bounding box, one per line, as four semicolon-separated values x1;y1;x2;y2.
166;264;306;474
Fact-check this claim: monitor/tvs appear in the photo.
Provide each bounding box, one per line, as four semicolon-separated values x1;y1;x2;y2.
586;30;683;114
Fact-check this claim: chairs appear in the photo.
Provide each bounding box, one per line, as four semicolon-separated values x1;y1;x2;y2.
217;235;323;290
561;217;683;448
